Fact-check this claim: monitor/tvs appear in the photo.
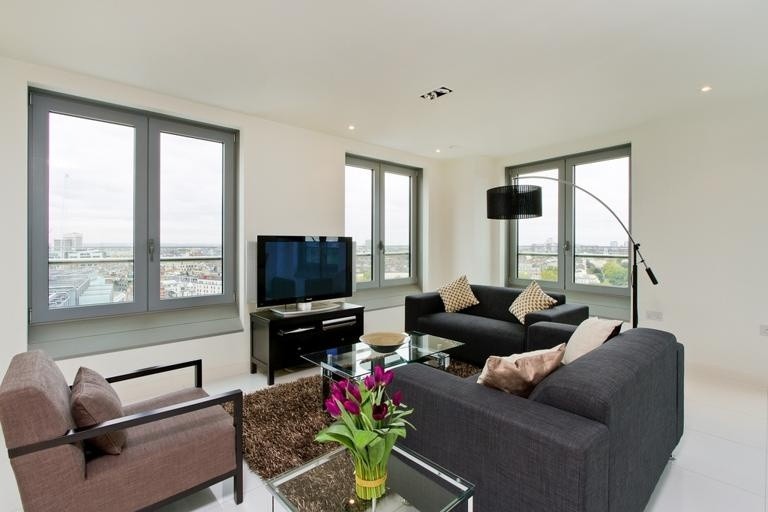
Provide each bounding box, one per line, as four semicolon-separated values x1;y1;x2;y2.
257;235;352;315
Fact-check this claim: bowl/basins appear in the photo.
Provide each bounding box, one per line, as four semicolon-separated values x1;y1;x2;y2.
359;332;410;353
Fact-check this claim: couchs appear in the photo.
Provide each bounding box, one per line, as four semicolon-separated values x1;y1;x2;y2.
0;349;243;512
404;284;590;362
385;321;684;511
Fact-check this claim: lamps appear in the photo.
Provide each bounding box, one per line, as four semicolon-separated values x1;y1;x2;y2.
486;168;658;328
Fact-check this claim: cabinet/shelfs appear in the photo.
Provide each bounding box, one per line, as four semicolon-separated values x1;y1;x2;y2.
250;301;366;385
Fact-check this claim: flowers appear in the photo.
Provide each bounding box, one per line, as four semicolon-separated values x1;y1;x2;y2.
312;365;417;500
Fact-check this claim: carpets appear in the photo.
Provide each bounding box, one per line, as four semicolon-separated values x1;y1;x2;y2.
217;349;482;512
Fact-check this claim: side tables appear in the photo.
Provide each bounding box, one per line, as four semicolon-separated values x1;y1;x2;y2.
267;442;477;512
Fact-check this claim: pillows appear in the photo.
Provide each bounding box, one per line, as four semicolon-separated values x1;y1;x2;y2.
70;366;127;456
560;316;623;366
508;279;558;325
437;273;479;313
476;342;566;395
